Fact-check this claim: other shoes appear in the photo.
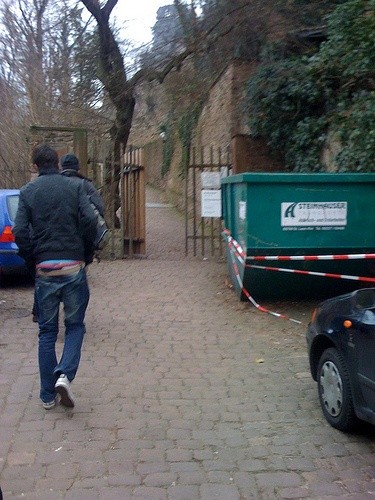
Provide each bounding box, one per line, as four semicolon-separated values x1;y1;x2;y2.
43;394;58;410
32;315;38;322
54;378;75;408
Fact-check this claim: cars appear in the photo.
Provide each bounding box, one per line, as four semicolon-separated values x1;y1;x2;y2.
305;289;375;428
0;188;36;273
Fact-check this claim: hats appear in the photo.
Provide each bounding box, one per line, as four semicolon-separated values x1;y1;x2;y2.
60;153;80;170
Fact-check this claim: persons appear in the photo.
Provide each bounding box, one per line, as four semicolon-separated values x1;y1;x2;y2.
31;152;114;335
12;143;99;411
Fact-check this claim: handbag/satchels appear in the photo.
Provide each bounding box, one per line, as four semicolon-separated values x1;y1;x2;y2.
80;179;112;251
